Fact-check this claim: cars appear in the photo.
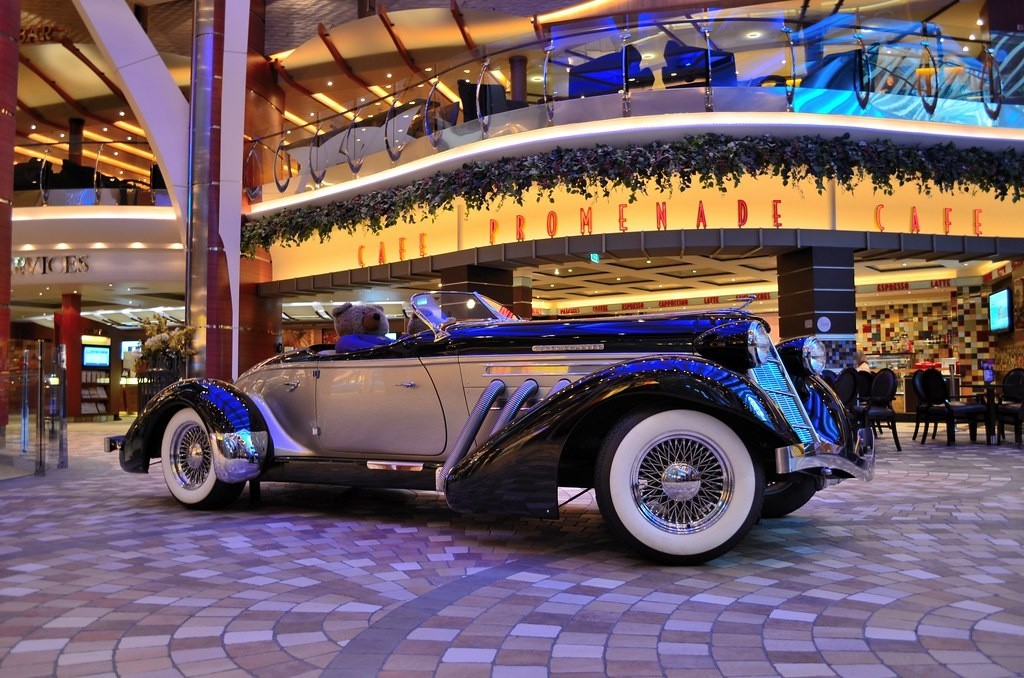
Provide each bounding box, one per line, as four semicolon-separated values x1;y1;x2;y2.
102;291;875;566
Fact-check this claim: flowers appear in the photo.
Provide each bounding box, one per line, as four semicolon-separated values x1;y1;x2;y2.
132;312;196;377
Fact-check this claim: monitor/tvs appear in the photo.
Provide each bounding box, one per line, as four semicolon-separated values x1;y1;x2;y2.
82;344;110;370
989;288;1013;334
121;340;143;360
385;331;399;340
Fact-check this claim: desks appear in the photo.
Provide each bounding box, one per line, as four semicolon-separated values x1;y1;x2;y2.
960;383;1010;447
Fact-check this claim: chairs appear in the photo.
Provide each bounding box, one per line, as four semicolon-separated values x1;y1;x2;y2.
457;42;880;124
13;157;168;207
818;367;1024;455
